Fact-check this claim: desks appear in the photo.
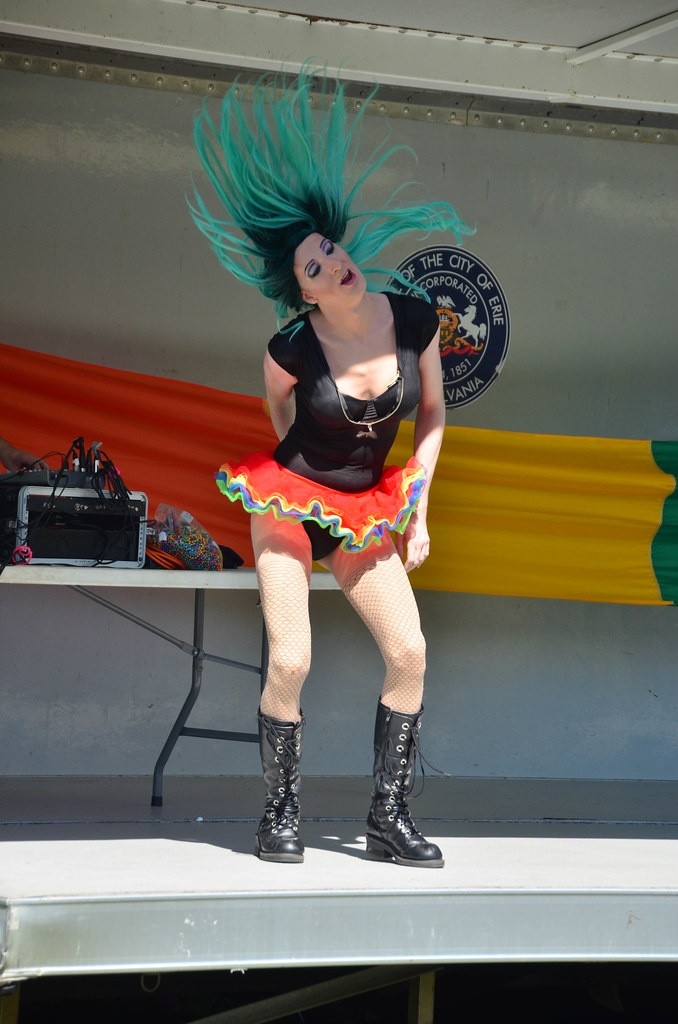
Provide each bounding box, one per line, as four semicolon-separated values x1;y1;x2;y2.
0;565;343;807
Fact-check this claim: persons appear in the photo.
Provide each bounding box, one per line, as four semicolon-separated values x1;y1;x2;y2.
183;58;477;868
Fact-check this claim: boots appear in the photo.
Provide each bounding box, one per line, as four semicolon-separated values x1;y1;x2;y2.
364;696;442;870
252;703;304;862
